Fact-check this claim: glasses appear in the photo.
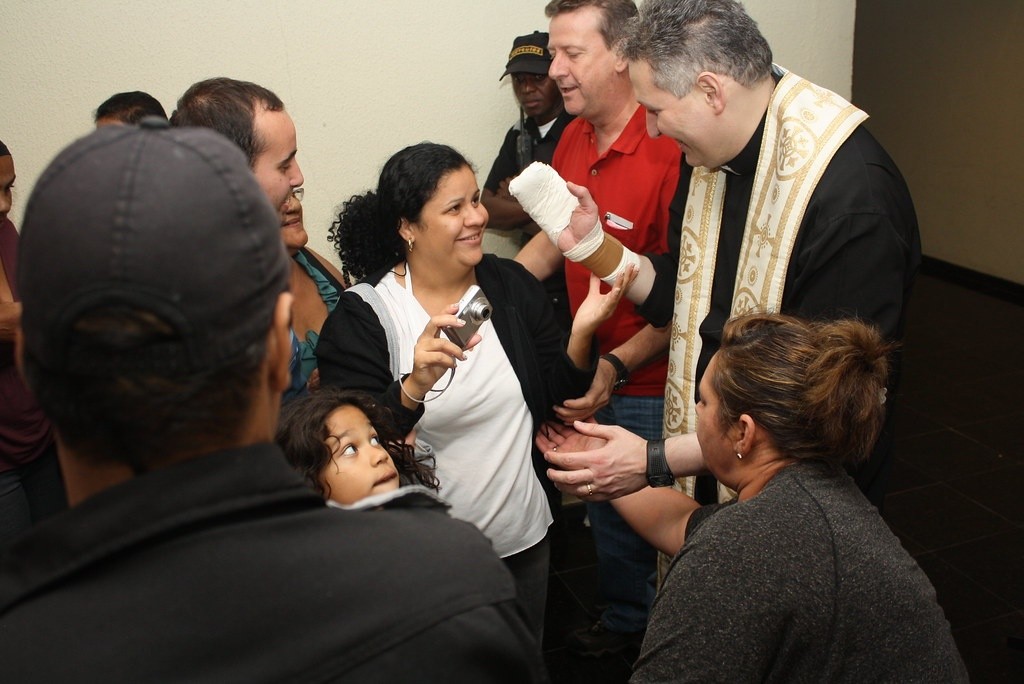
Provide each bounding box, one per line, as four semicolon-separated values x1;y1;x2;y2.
284;187;304;201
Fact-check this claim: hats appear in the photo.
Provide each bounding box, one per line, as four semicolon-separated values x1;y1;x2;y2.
16;115;288;373
498;30;552;81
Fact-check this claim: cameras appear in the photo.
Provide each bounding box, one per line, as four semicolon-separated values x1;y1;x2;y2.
441;284;493;349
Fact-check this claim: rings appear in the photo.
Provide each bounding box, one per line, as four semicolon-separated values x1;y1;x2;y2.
553;446;558;452
587;483;592;496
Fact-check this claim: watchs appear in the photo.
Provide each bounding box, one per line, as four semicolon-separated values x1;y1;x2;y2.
646;439;674;488
601;354;630;391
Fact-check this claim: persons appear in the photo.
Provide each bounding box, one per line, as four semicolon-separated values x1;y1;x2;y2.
632;313;970;683
545;1;921;505
169;77;350;390
280;385;440;506
0;115;552;683
95;91;167;125
316;142;639;654
482;30;571;322
515;0;681;659
0;142;64;539
